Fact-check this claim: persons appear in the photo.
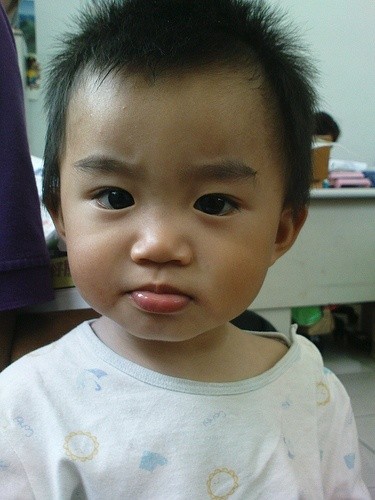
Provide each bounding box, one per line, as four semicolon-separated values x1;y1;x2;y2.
310;112;340;158
1;0;374;499
